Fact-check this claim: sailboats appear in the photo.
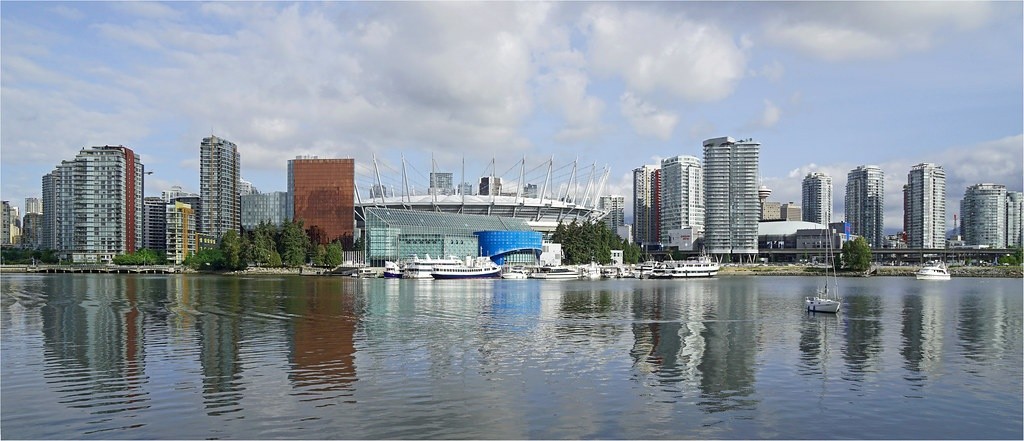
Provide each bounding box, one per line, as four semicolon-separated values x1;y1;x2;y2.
804;210;842;314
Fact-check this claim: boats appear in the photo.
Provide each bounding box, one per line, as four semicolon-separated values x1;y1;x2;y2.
501;270;527;280
601;256;721;279
383;252;502;280
529;265;578;278
914;266;951;281
